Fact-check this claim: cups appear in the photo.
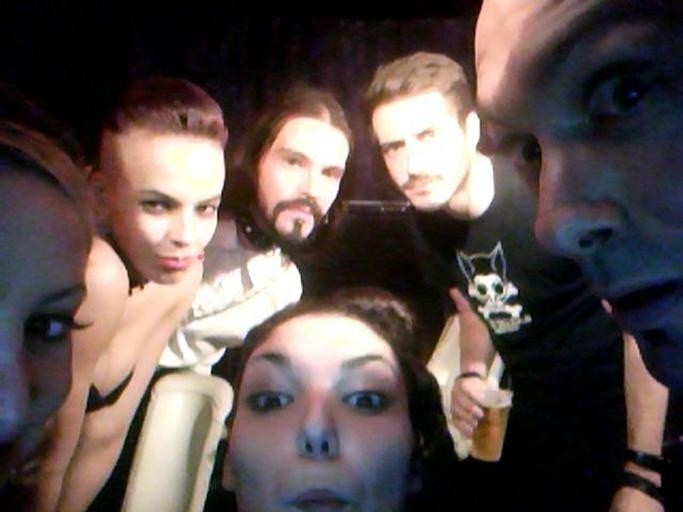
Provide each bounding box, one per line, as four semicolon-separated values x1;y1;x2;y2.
469;389;516;462
